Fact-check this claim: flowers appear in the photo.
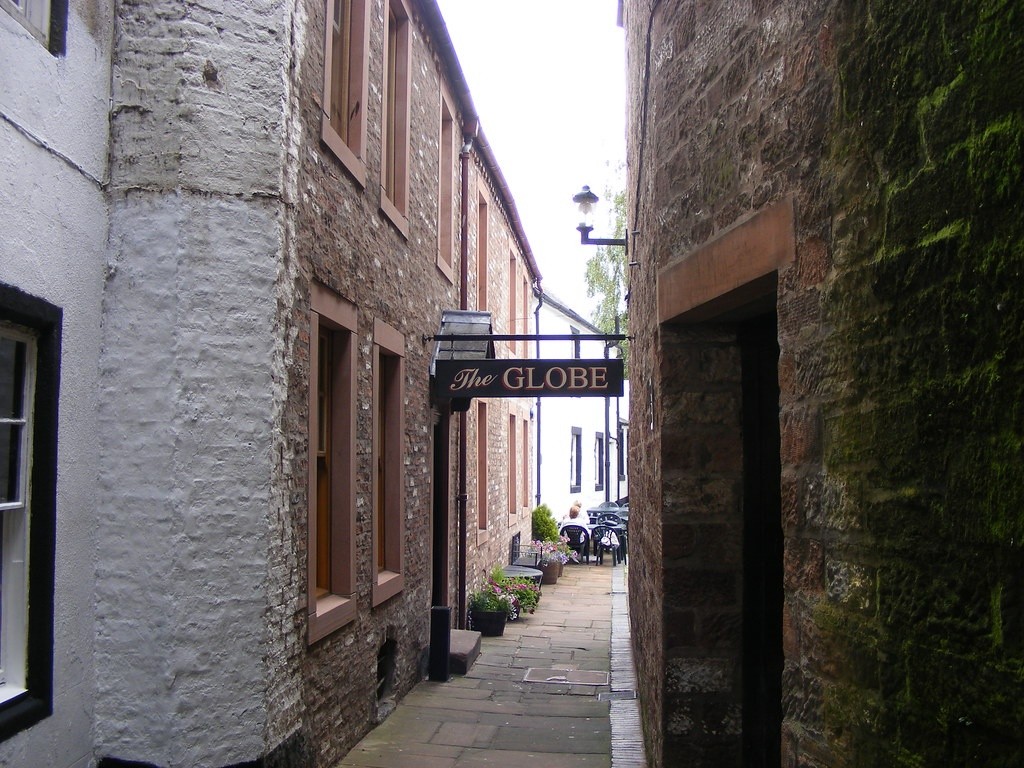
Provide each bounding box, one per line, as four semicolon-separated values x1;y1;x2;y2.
532;535;580;564
486;576;541;620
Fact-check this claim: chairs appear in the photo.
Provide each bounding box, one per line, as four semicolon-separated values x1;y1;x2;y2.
560;526;590;564
592;526;626;567
597;513;621;525
598;501;619;524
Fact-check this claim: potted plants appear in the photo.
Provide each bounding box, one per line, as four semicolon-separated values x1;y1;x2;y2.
469;592;510;636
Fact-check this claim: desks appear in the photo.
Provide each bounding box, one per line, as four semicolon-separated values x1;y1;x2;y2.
586;507;629;526
587;524;625;562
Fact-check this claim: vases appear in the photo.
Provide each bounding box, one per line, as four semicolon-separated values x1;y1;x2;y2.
507;600;520;621
536;562;560;584
559;562;563;577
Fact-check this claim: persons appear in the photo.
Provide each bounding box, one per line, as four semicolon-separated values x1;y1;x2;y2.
559;500;590;564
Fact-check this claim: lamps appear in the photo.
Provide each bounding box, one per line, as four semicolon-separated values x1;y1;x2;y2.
575;185;628;255
